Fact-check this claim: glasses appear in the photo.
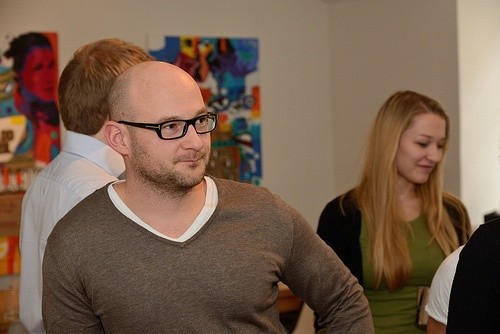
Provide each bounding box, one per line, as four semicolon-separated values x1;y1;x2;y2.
117;111;218;141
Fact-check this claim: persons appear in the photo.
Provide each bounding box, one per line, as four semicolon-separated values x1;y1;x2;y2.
20;38;158;334
424;213;500;334
41;60;374;334
315;90;471;334
